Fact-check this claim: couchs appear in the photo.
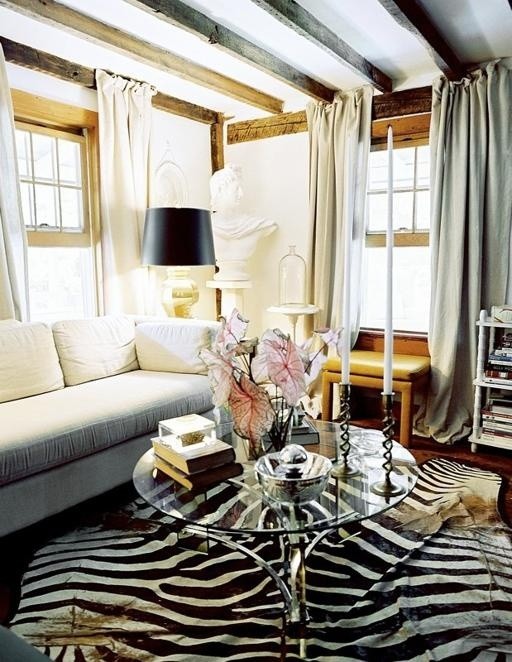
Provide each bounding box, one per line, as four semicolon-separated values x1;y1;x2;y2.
0;318;223;537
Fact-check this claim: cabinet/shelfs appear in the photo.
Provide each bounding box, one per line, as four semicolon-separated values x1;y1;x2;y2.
468;310;512;453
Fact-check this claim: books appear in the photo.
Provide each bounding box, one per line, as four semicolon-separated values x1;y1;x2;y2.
480;393;512;447
154;454;244;491
264;414;320;446
292;420;310;435
150;436;237;475
483;333;512;387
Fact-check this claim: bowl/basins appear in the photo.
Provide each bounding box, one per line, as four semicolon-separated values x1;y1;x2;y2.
253;443;334;507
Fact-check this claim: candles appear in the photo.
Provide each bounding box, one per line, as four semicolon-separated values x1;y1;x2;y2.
341;136;351;381
383;125;393;390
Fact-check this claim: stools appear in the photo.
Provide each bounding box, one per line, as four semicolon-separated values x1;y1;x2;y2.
321;349;431;446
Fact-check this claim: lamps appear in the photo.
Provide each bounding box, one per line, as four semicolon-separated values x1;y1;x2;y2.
141;208;215;318
